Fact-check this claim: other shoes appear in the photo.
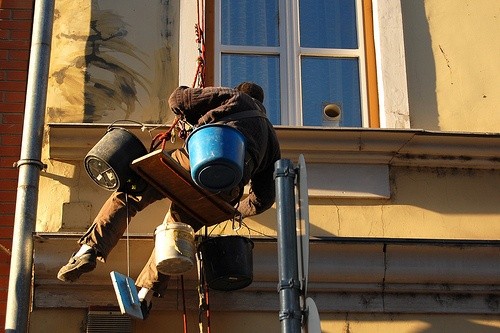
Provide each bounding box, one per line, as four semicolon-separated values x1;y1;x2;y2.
138;298;153;320
56;249;96;284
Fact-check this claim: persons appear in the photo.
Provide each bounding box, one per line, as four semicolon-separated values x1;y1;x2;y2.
57;81;282;299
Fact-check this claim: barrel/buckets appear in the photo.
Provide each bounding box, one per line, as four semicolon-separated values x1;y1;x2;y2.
153;210;195;276
183;119;246;192
83;119;154;192
197;219;254;292
